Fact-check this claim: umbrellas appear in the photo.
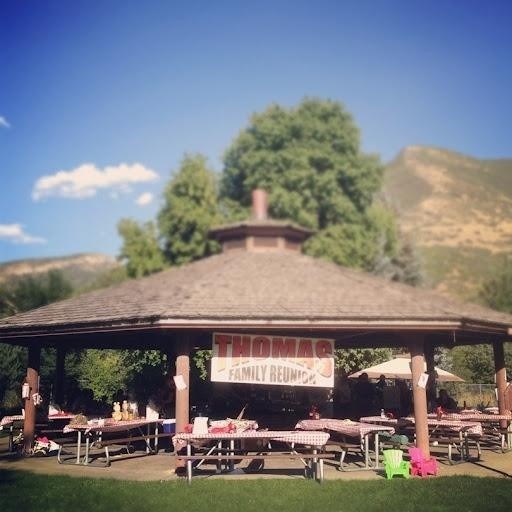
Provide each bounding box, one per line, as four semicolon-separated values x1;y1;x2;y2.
346;357;465;383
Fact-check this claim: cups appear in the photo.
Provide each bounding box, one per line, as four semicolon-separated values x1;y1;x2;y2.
99;419;104;427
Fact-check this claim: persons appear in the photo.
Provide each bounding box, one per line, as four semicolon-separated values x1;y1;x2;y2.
59;371;461;451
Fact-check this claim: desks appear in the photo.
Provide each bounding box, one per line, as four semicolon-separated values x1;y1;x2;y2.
4;415;163;468
295;415;512;471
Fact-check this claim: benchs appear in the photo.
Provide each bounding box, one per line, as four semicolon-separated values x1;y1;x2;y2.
98;430;512;487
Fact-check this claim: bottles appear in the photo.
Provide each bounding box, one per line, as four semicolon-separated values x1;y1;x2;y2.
380;409;386;420
436;407;443;421
